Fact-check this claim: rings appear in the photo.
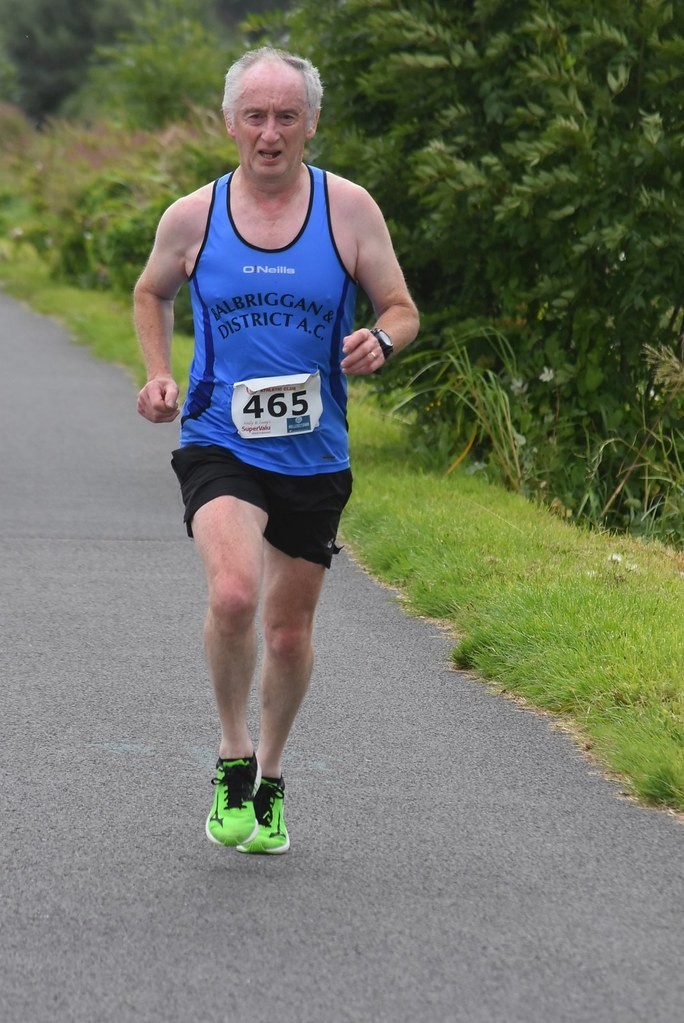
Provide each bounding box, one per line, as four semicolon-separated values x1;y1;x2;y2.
371;352;376;358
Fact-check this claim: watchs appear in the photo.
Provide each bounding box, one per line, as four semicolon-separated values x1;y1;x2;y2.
370;327;394;359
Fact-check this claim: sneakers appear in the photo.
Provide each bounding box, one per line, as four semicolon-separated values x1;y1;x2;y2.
237;772;291;854
205;750;257;846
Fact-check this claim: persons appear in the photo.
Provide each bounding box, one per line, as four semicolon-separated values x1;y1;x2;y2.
134;46;419;851
